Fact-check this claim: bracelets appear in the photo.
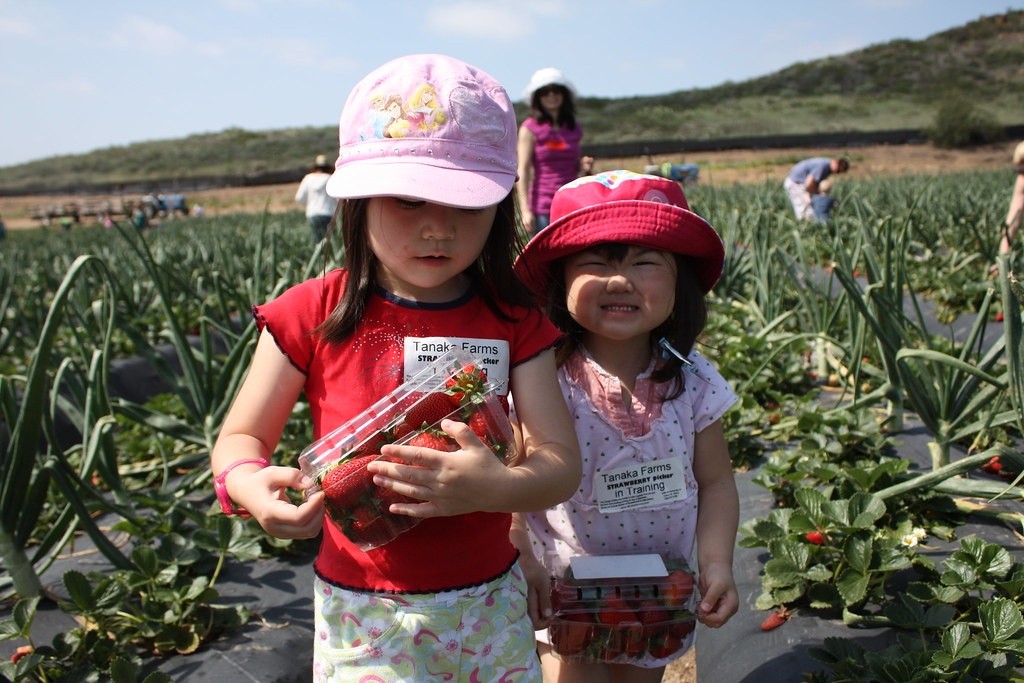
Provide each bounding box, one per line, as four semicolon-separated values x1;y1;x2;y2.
214;457;270;517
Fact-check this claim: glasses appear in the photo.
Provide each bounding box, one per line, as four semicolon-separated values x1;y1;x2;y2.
539;86;562;97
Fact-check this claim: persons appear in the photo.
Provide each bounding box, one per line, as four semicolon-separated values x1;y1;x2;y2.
516;69;595;235
781;157;850;223
645;163;700;183
211;55;583;683
293;155;339;252
512;168;741;683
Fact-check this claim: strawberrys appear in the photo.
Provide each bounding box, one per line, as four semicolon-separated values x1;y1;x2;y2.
996;312;1003;321
804;532;826;544
12;645;34;664
760;611;786;630
548;554;697;661
763;334;873;425
989;457;1002;471
314;362;511;546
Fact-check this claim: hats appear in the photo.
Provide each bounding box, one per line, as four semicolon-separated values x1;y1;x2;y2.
325;53;518;209
522;68;577;108
306;155;335;173
512;170;725;311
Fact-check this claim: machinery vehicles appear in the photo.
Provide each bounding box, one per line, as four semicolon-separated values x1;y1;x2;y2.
125;193;190;221
640;146;701;184
